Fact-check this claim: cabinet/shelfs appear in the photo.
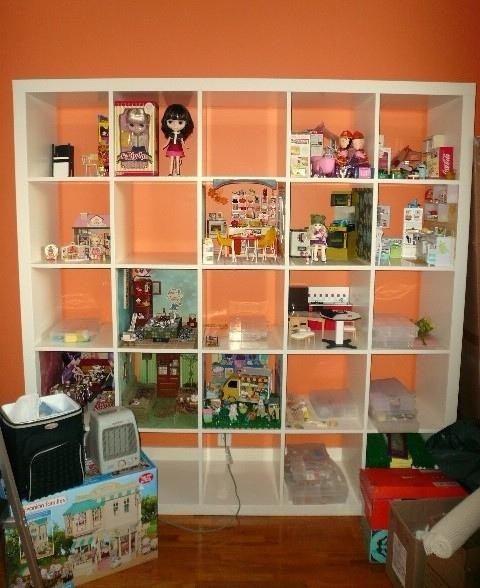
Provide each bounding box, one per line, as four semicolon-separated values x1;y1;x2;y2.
11;77;477;517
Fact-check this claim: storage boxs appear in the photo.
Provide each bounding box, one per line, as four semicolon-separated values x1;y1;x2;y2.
358;468;480;588
